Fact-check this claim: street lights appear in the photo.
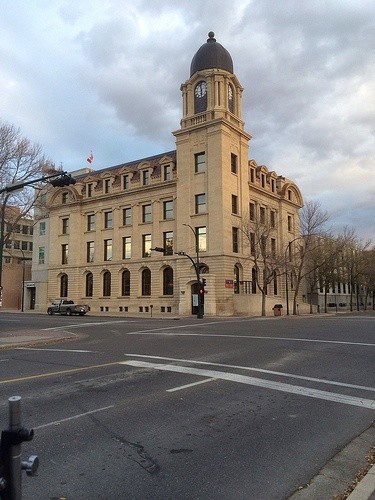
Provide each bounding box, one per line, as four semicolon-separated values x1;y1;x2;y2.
182;222;204;320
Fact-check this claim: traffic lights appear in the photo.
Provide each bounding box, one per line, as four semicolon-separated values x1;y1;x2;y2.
50;174;76;188
200;289;203;294
178;251;186;256
201;277;206;286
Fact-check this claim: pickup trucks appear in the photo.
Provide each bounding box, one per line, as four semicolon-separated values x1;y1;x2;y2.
46;298;89;316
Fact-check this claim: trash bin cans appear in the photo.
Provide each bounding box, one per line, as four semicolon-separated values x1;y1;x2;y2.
274;305;284;316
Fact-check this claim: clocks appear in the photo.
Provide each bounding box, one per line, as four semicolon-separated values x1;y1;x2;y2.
195;81;207;98
228;86;233;100
